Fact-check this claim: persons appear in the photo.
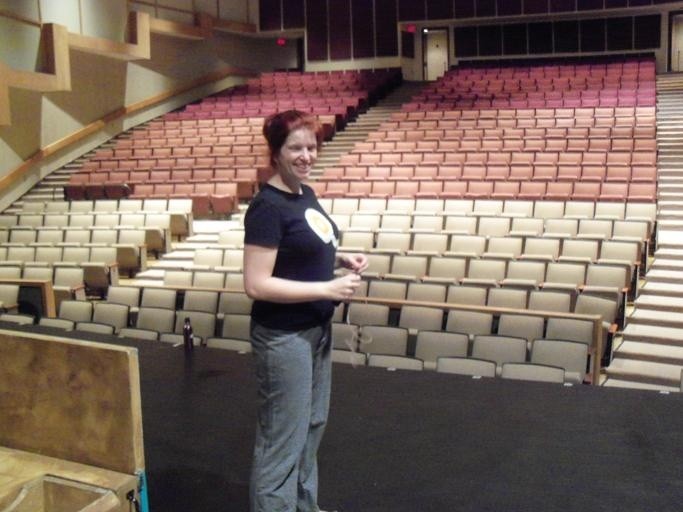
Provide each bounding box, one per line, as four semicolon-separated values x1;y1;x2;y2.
240;107;370;512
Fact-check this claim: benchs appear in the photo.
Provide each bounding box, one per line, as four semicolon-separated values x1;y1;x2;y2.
1;60;658;384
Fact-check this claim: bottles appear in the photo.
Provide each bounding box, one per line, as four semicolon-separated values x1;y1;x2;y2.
183;317;194;355
